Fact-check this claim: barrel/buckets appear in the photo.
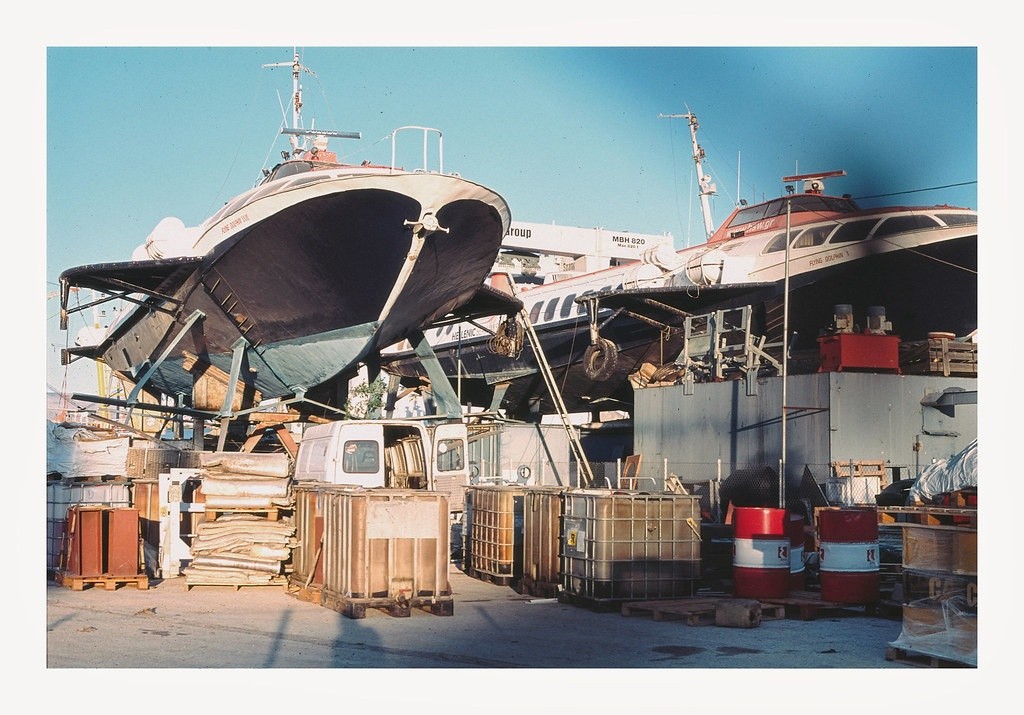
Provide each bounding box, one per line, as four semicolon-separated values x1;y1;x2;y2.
926;330;956;362
818;509;880;603
732;506;792;598
790;513;806;592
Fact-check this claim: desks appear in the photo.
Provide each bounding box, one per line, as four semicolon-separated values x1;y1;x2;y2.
815;333;904;374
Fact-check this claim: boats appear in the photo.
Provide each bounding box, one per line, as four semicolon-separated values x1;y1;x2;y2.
372;101;977;420
58;46;523;423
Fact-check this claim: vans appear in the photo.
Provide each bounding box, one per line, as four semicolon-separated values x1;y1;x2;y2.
295;419;471;515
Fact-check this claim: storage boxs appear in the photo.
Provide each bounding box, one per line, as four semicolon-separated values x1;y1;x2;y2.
293;484;705;613
